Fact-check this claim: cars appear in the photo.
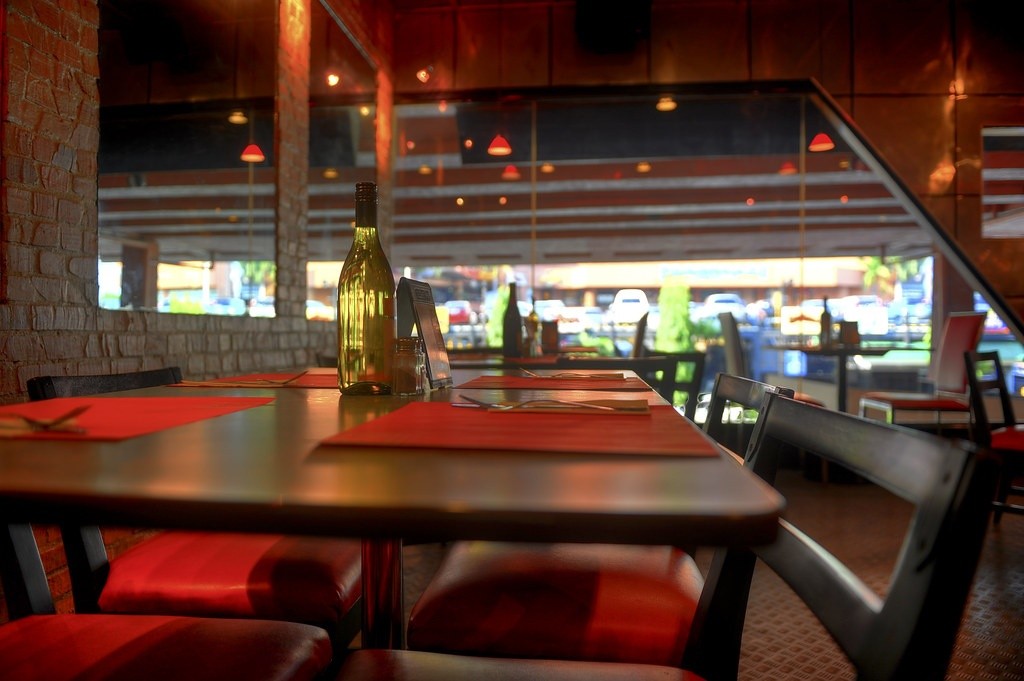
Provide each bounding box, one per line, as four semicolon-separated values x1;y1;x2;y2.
781;295;930;328
207;295;335;322
534;288;657;332
687;293;774;328
444;299;476;326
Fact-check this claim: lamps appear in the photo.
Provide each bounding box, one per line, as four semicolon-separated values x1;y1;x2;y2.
778;156;798;174
502;112;522;181
487;97;513;154
240;102;264;162
226;22;249;123
807;116;834;152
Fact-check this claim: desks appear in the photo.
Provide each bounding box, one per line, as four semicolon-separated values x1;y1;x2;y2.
447;351;602;368
0;368;787;654
766;342;895;411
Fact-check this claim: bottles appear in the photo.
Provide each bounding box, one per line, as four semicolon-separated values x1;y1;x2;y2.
502;282;522;358
339;182;395;394
525;300;558;358
819;297;833;348
391;337;427;397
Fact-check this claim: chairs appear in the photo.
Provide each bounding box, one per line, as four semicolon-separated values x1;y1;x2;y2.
0;310;1024;681
